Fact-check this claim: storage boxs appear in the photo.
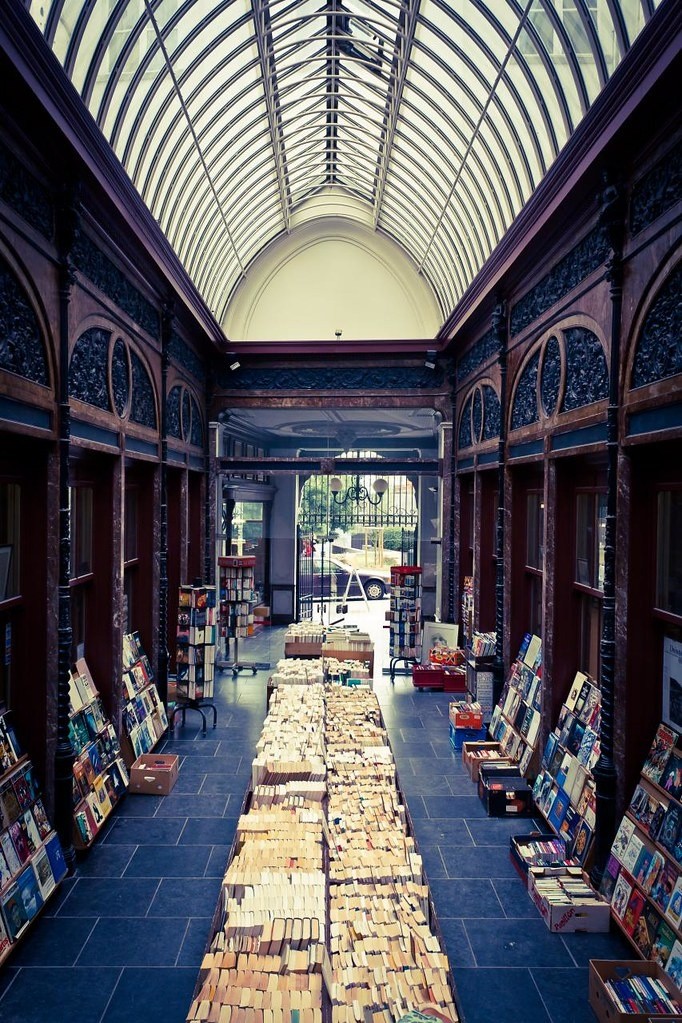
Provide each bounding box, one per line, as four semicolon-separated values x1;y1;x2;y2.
129;754;178;794
207;643;465;1023
510;833;612;933
449;708;534;817
588;958;682;1022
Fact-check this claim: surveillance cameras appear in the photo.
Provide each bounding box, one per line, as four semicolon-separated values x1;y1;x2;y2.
335;329;342;336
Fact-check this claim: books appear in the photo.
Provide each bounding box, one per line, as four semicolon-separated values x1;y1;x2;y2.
170;556;450;1023
0;709;68;965
450;575;603;930
601;723;682;1019
68;648;172;846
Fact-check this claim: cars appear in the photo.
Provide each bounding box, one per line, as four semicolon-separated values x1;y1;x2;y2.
298;555;392;601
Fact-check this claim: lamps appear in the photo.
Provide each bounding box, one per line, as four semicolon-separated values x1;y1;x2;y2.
331;474;389;505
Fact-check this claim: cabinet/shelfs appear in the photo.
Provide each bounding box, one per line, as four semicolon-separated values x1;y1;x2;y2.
1;711;70;967
218;554;258;677
123;634;167;761
387;566;424;680
66;665;128;849
169;579;218;734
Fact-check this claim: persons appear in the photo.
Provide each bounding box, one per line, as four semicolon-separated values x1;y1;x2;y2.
302;538;312;557
